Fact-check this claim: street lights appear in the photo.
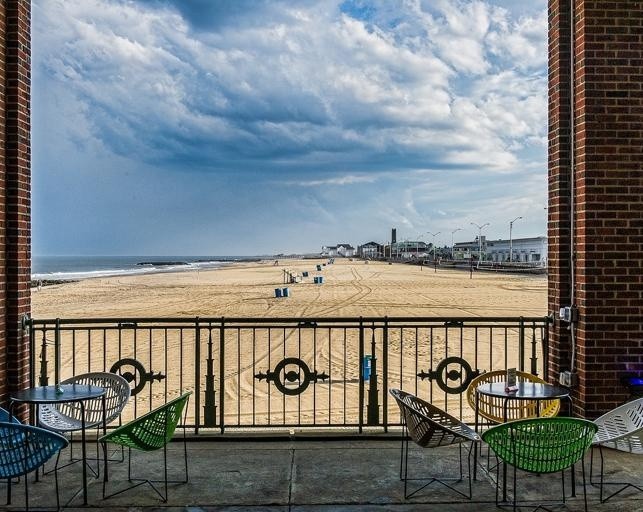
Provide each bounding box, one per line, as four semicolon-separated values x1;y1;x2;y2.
427;232;441;260
510;217;523;262
452;228;462;257
471;222;490;261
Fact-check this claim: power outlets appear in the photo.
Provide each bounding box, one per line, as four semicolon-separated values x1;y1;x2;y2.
562;370;571;388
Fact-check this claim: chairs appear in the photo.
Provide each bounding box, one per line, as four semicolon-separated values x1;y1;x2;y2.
0;370;194;512
388;366;643;512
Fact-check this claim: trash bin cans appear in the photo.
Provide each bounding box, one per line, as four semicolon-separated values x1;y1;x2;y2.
314;277;322;283
275;288;288;297
317;265;321;271
364;355;372;380
323;264;326;266
303;272;308;276
